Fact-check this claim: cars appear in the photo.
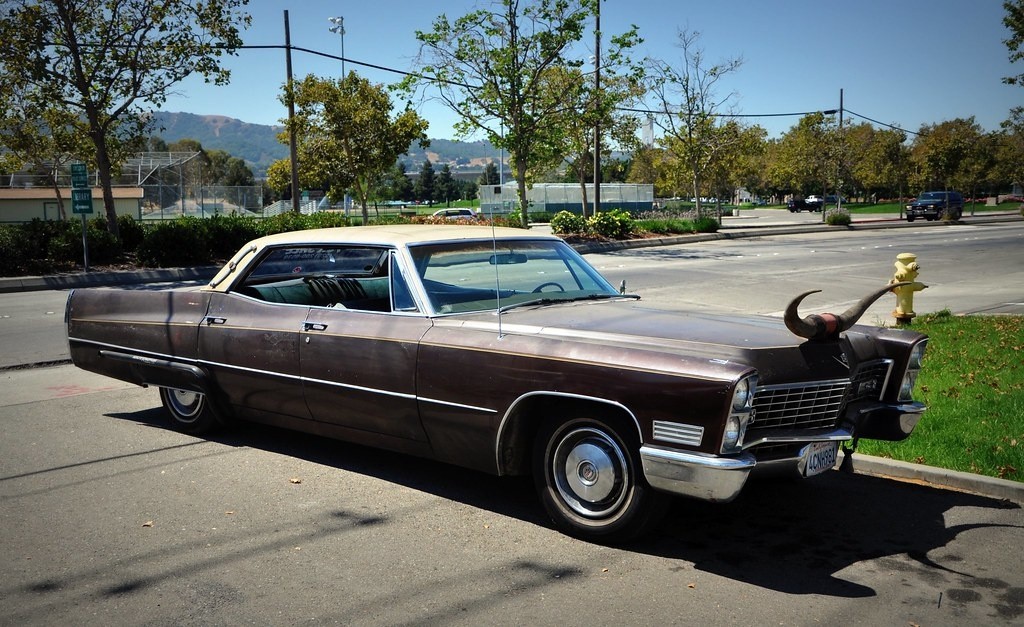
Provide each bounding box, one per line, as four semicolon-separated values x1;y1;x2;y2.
825;195;846;205
66;223;930;545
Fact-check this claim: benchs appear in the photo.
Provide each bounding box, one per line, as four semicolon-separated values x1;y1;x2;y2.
246;275;390;311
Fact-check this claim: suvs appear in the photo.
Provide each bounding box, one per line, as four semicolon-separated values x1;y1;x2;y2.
428;208;478;222
905;191;964;223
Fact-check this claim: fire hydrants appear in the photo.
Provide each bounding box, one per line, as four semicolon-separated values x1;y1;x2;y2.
886;253;929;327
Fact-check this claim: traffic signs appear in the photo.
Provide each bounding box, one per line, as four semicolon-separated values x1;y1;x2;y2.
71;188;94;215
71;163;87;188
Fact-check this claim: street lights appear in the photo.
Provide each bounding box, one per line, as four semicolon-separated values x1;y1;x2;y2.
328;15;346;83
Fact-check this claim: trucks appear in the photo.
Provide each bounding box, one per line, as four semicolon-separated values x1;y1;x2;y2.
788;197;821;214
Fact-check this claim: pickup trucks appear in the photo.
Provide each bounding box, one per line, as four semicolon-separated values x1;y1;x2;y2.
805;195;824;204
